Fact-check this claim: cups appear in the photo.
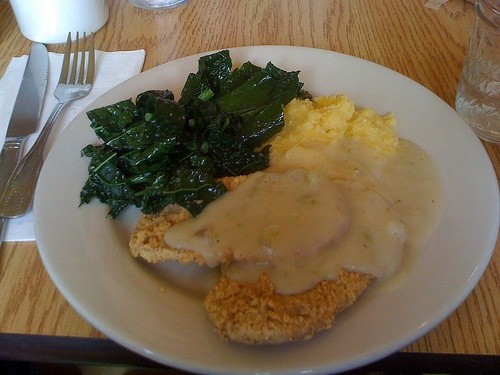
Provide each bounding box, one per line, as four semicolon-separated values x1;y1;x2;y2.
7;2;108;41
450;2;499;145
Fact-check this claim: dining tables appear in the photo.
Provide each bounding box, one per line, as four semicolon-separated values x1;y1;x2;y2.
0;0;500;375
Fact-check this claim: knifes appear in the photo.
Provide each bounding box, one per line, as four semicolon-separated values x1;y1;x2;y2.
0;40;51;240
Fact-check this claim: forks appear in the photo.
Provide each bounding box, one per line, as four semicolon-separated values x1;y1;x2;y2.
2;31;96;222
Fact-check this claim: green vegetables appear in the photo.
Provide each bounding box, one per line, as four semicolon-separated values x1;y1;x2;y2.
76;49;314;219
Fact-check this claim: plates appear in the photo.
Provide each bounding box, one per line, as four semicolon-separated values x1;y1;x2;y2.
33;44;499;375
